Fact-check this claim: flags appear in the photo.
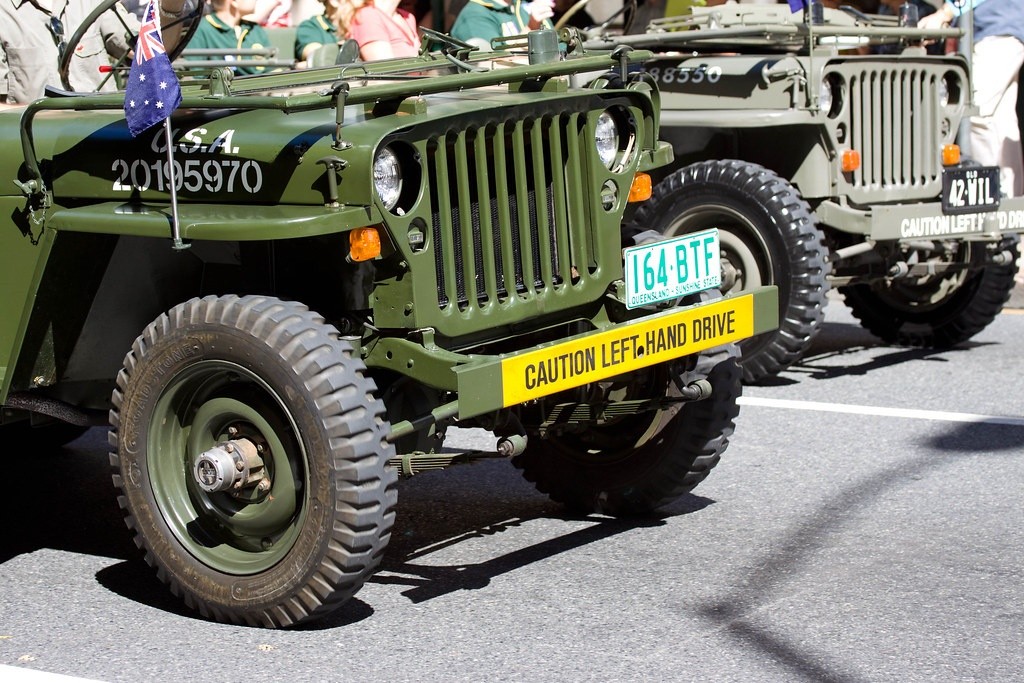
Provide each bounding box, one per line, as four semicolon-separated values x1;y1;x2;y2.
123;0;184;137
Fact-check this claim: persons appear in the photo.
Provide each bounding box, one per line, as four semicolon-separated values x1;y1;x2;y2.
0;0;563;87
917;0;1024;199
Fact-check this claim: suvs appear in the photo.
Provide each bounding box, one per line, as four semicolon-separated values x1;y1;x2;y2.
0;0;781;629
490;0;1024;387
